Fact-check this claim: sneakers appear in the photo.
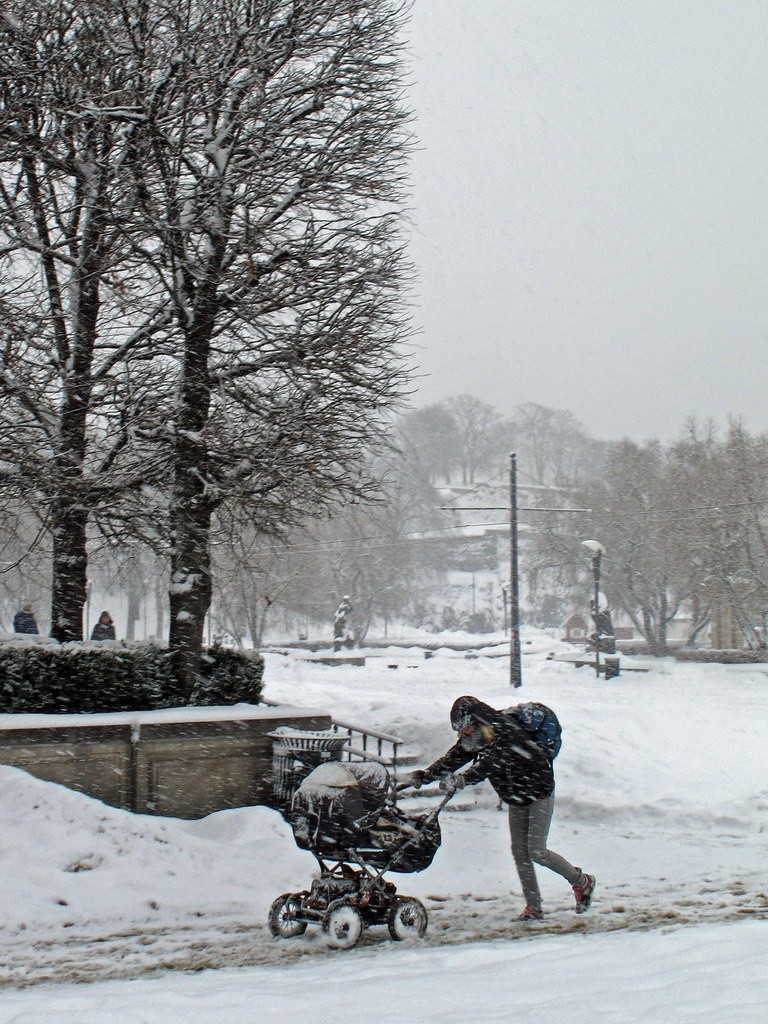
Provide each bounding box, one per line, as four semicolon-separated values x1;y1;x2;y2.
511;906;544;922
573;867;596;914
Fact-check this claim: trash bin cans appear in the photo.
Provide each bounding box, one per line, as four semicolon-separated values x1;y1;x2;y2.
605;658;621;682
268;730;352;821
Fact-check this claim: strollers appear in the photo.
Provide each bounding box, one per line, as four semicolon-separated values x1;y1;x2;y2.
267;761;461;951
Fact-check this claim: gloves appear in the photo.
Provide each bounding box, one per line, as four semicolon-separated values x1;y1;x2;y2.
406;770;431;790
439;774;465;791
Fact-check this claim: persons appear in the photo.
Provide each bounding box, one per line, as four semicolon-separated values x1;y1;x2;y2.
91;611;116;640
14;604;39;634
408;696;596;922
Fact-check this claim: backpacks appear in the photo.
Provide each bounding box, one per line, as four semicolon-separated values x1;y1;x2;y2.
501;703;561;761
